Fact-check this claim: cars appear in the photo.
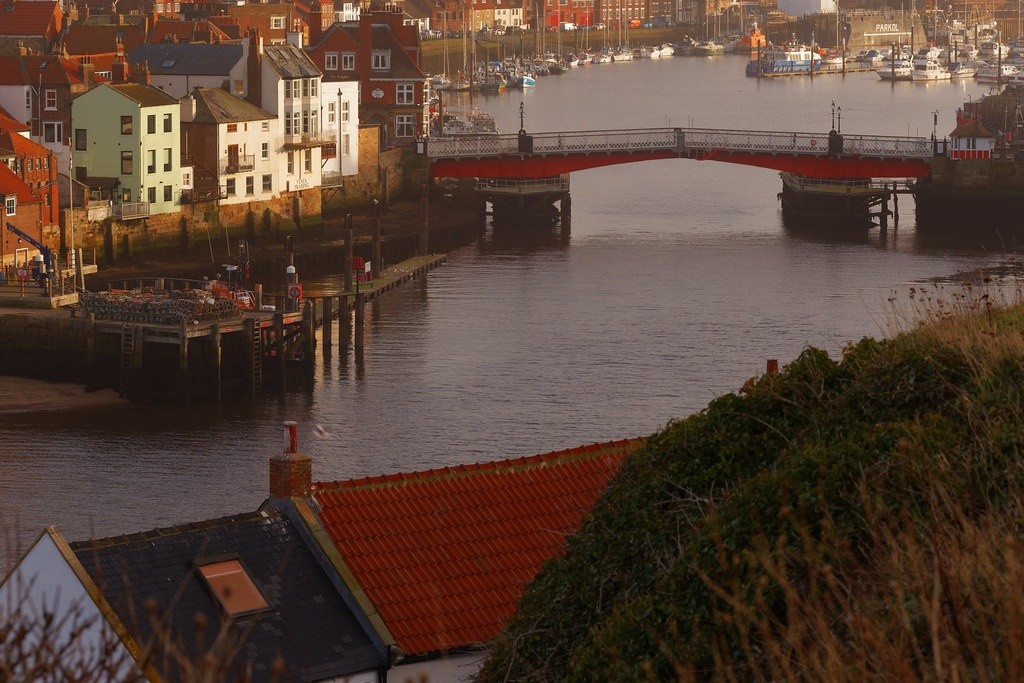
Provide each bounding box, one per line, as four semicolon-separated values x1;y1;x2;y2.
421;11;722;39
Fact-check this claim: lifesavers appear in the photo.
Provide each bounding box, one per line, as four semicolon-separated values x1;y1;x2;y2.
811;140;817;146
290;286;300;299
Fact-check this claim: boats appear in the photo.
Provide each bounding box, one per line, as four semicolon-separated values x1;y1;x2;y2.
821;0;1024;65
977;63;1021;83
876;59;915;80
431;0;739;95
746;47;822;77
910;59;951;81
